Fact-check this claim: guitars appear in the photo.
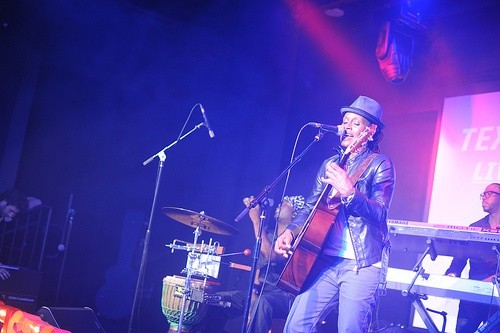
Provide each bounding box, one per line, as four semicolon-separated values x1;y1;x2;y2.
276;124;377;296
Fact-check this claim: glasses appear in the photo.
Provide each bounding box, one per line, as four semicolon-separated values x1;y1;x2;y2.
480;191;500;198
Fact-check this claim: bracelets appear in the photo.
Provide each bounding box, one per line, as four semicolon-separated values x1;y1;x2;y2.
340;196;353;204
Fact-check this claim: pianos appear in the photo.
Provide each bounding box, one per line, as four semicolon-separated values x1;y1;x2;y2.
384;219;500;333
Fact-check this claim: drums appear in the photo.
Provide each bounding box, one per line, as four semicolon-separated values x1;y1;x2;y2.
161;274;221;333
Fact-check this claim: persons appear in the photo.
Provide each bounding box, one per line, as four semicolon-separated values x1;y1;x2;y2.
203;196;305;333
0;188;29;280
445;183;500;333
274;96;396;333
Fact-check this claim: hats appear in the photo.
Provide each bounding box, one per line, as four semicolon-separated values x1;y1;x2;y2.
282;195;304;215
340;95;385;129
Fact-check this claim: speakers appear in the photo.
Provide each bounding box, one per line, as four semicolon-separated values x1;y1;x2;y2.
36;306;106;333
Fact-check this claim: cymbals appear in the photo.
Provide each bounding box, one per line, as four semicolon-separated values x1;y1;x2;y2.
161;206;240;236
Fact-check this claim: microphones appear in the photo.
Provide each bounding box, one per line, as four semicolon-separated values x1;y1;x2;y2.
308;122;345;136
427;239;437;261
200;104;215;138
